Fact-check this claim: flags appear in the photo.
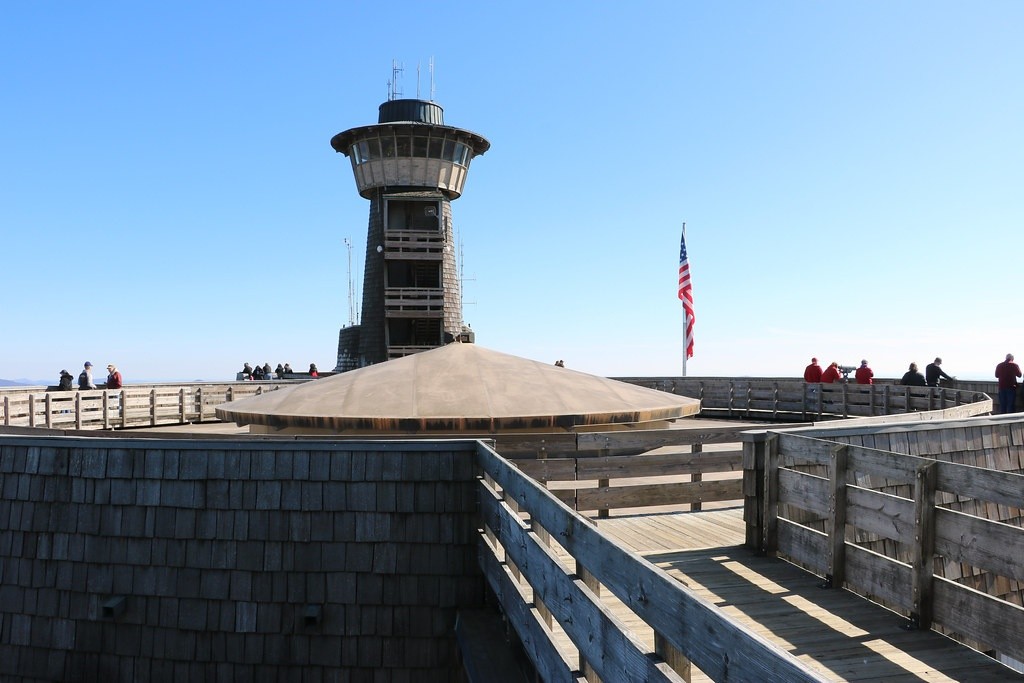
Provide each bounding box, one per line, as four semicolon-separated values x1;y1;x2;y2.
678;225;696;359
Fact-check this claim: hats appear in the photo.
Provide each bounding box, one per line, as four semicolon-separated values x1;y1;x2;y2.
106;364;115;369
812;358;818;362
84;362;93;366
936;358;942;364
861;359;868;364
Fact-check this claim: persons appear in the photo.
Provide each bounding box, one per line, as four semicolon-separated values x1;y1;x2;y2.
555;360;564;367
901;357;956;410
58;370;72;413
309;364;319;377
855;360;873;405
804;358;840;405
995;354;1022;414
106;364;122;410
243;363;292;381
74;362;100;422
